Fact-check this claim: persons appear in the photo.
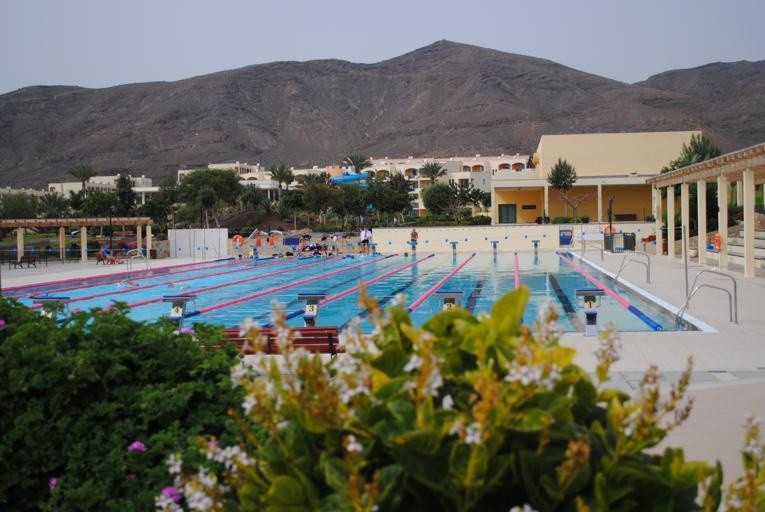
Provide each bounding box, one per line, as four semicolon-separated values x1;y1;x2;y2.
286;249;293;256
363;227;371;245
359;227;365;243
298;233;335;258
71;241;76;248
103;247;115;264
411;229;418;249
269;234;279;258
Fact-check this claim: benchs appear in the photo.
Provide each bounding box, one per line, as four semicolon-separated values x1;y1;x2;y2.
13;255;36;269
96;254;108;264
198;326;340;355
